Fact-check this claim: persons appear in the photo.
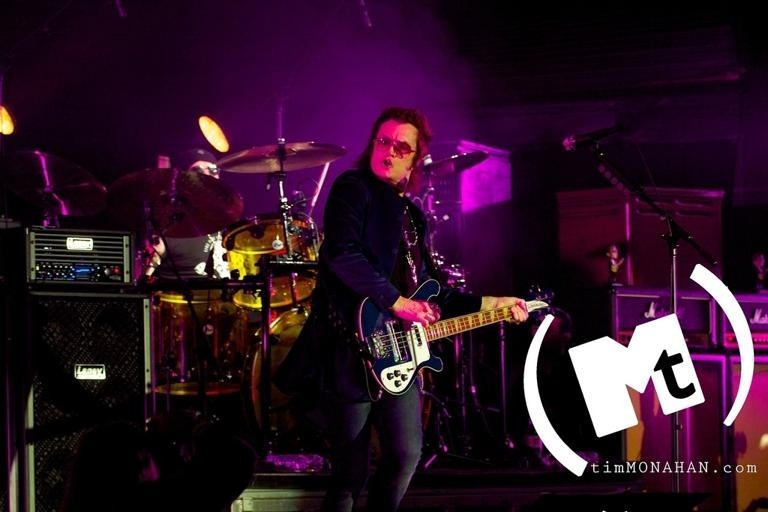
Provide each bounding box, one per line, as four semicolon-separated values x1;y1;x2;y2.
274;106;531;512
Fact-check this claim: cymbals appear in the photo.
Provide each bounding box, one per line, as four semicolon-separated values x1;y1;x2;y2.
217;140;346;176
104;166;245;238
1;151;107;215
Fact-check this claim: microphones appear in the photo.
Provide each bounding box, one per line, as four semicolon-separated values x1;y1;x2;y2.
562;123;619;151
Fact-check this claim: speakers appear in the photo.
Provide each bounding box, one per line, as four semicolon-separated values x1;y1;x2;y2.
18;292;186;512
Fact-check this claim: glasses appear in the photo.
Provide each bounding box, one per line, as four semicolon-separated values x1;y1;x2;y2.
373;137;417;154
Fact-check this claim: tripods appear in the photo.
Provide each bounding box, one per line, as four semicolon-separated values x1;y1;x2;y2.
420;312;538;474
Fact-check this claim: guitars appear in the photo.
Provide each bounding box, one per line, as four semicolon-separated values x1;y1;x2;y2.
346;277;556;397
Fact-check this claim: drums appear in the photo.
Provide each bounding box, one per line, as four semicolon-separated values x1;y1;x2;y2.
146;273;253;397
222;219;318;310
236;303;312;430
